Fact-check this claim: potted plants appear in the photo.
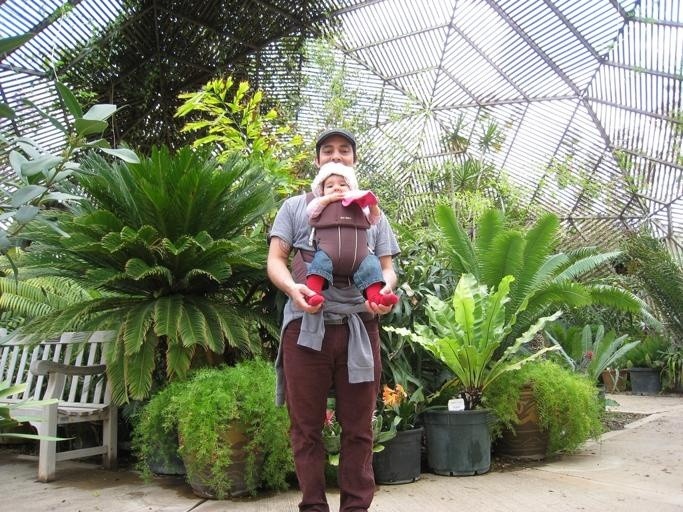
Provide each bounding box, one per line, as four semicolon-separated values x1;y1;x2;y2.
0;147;667;499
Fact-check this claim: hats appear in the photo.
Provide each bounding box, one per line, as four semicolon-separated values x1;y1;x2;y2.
311;161;359;198
316;129;356;151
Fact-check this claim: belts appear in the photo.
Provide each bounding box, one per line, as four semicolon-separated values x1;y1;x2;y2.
325;312;375;326
